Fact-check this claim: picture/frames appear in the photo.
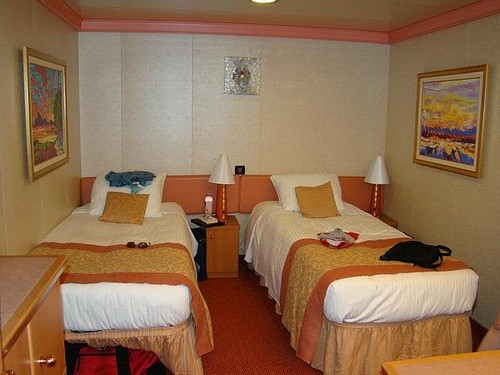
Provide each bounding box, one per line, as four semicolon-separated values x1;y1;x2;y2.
413;64;488;178
23;45;69;181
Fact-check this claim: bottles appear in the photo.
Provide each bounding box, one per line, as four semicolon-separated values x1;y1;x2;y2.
204;193;213;216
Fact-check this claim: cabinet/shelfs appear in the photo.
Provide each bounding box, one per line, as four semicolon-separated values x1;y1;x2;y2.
0;254;70;375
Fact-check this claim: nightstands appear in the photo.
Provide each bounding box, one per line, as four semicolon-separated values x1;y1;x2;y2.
204;215;241;279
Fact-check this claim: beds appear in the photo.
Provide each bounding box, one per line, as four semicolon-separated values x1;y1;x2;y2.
238;173;479;375
25;173;241;375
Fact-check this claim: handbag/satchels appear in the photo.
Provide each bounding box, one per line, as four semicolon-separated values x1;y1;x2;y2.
380;241;452;271
73;344;175;375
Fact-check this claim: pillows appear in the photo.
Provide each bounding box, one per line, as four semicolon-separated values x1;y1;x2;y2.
88;170;168;225
269;172;345;219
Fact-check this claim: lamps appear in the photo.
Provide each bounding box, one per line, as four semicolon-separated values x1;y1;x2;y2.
363;155;390;218
208;154;236;223
